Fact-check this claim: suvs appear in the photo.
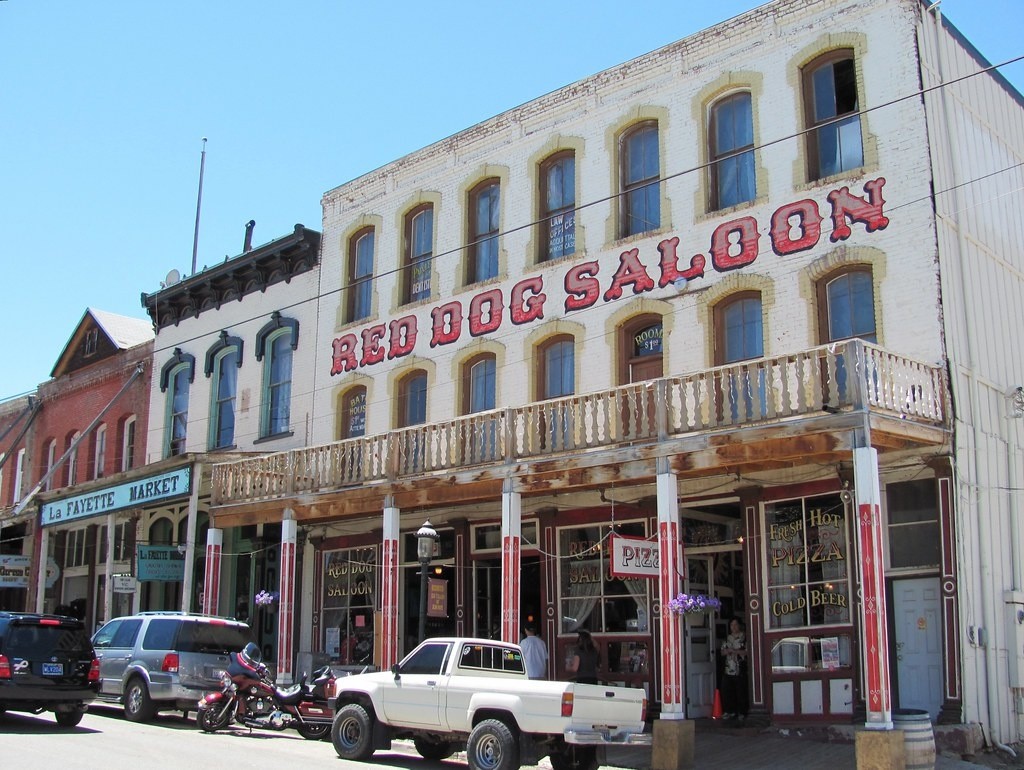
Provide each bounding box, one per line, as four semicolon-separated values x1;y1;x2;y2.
91;611;259;723
0;612;103;727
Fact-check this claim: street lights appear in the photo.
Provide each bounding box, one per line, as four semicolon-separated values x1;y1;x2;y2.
417;517;441;645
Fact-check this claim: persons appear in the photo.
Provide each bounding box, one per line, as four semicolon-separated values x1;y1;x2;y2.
572;630;600;684
519;622;549;680
720;618;749;722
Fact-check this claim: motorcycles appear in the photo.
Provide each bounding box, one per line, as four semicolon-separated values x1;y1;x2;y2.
196;641;353;740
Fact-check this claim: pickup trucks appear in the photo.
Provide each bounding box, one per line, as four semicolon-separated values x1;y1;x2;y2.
770;638;822;673
327;637;647;770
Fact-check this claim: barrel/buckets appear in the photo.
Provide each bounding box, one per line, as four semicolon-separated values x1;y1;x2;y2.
892;708;937;770
